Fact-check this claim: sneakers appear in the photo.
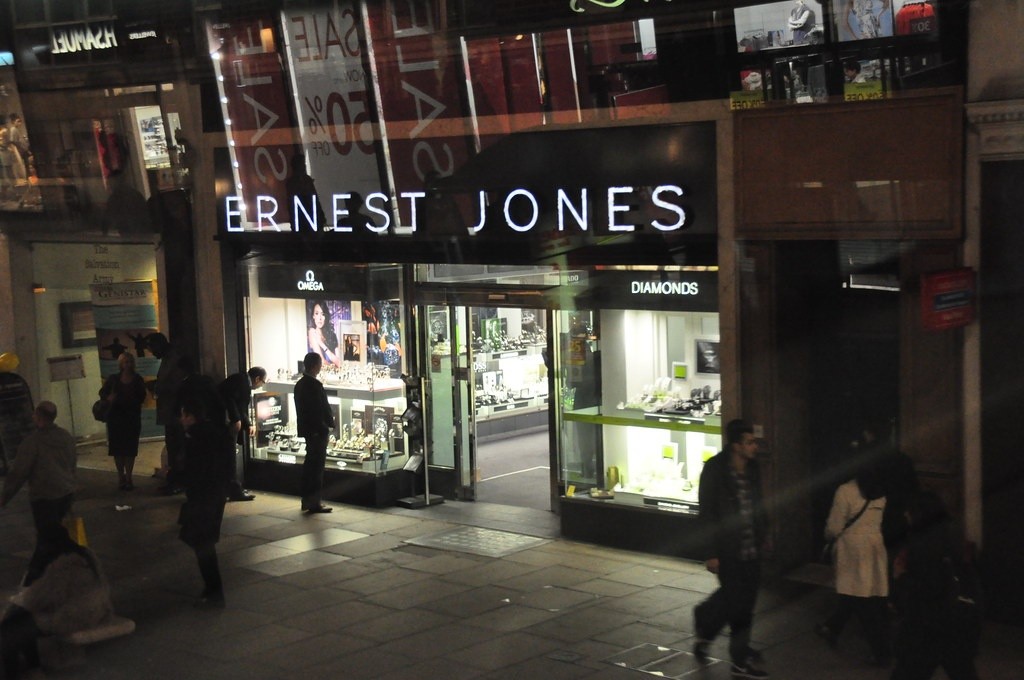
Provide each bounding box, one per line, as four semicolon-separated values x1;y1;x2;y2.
693;605;712;665
731;663;768;680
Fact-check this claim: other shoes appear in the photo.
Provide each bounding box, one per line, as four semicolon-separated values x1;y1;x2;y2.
11;592;30;609
193;596;225;608
811;620;836;646
158;483;182;495
230;494;255;501
119;478;133;491
302;503;332;513
86;555;104;576
865;655;890;668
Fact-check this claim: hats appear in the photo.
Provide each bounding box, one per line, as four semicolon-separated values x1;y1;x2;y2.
145;332;169;347
251;368;265;383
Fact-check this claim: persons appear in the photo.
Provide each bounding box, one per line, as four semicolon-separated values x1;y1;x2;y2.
293;351;334;513
0;353;36;476
345;336;358;359
98;353;147;492
307;299;341;367
142;333;236;608
217;367;267;500
845;61;866;83
842;0;890;39
811;413;927;667
487;319;500;339
692;421;772;680
0;524;113;680
788;0;810;44
0;115;26;209
0;400;88;536
888;503;981;680
10;113;31;202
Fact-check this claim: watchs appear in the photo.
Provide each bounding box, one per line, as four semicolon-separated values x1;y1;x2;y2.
432;317;445;341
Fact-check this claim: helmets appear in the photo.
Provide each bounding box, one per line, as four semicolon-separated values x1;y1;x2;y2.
0;352;19;372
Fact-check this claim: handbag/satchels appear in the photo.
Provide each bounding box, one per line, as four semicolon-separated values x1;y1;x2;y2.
92;399;114;421
820;543;832;564
61;509;87;547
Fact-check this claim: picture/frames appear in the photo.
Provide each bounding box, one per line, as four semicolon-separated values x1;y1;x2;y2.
694;339;720;377
340;320;367;365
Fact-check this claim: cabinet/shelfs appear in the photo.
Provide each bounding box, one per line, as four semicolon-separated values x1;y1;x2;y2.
472;346;549;444
241;366;411;511
558;405;721;560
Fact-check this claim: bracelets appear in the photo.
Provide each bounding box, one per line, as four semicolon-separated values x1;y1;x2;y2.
324;347;329;352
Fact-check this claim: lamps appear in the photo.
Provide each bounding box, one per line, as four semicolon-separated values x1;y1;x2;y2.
258;20;275;53
34;283;46;293
